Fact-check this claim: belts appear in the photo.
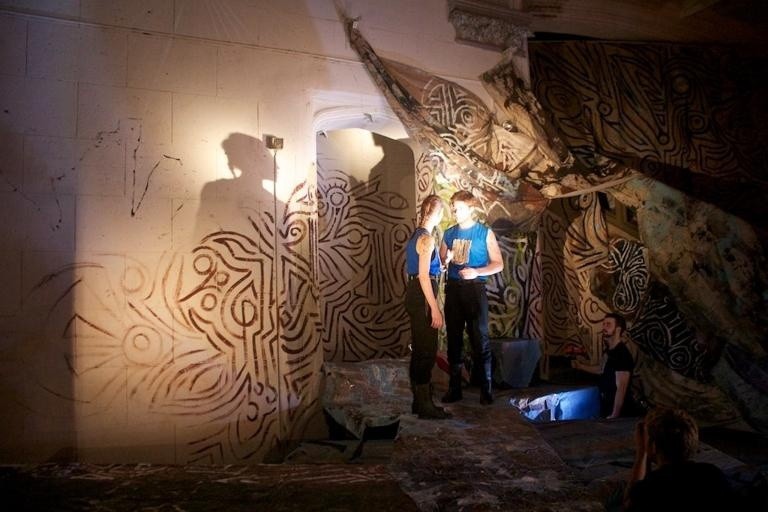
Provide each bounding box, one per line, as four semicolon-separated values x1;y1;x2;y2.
410;274;435;280
446;279;485;286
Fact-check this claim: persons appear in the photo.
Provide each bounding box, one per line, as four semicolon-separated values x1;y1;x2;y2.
522;314;633;420
404;195;453;420
439;189;504;404
615;408;743;512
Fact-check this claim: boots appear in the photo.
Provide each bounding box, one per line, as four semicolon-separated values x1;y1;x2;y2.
480;380;496;404
410;384;452;419
441;378;463;404
